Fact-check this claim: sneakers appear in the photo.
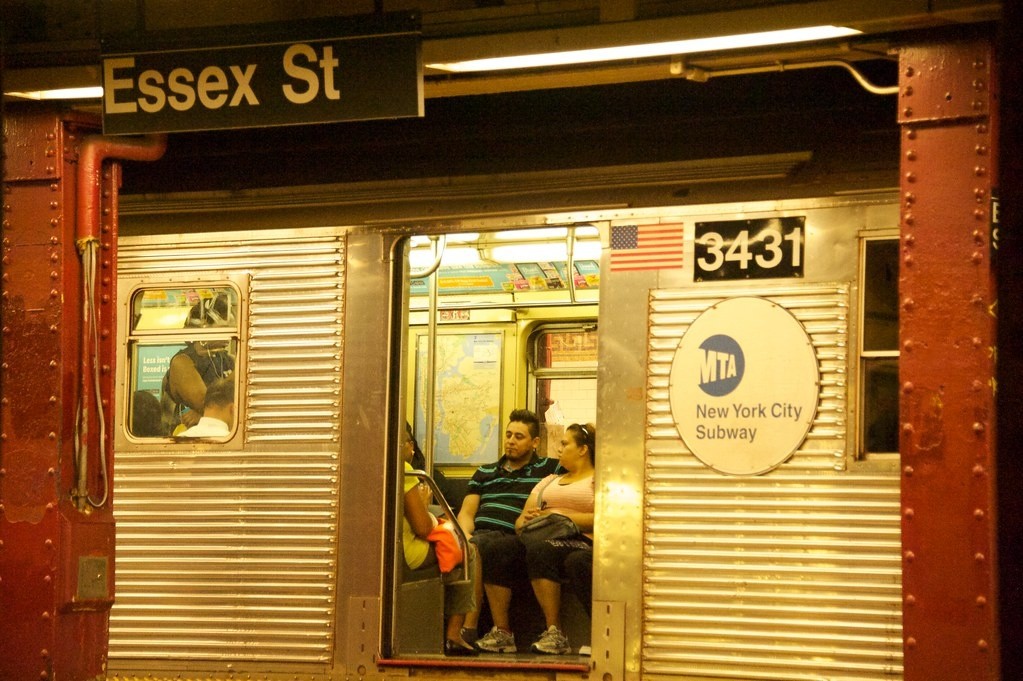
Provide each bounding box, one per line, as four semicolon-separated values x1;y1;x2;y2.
474;625;517;653
530;625;572;655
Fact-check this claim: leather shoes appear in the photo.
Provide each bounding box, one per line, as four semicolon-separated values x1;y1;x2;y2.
444;638;479;656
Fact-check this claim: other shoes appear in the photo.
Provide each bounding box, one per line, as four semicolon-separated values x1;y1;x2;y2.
445;627;478;650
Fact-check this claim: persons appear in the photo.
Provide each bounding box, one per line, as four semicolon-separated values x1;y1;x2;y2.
461;409;570;645
164;298;235;436
132;390;161;437
564;551;593;656
403;418;479;656
176;379;234;436
475;423;595;655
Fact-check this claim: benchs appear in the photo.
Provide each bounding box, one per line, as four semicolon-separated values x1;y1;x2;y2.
399;543;442;584
444;477;469;517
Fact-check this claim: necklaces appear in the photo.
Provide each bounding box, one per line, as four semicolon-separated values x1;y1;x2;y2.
507;462;513;472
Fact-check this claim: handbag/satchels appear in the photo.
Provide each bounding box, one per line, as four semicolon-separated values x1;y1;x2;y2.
519;513;582;547
424;516;464;573
